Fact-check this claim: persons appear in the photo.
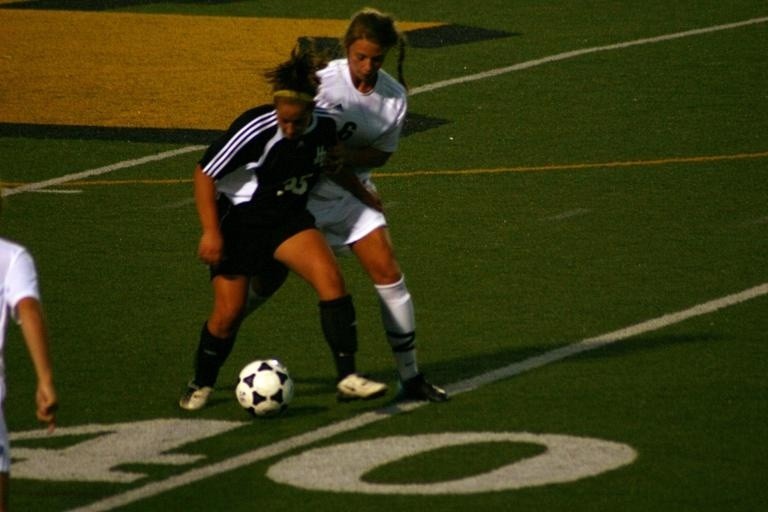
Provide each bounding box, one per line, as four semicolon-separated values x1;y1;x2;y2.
180;53;389;411
188;10;447;401
0;240;59;512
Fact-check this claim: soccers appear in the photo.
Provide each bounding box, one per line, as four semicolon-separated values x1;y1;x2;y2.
235;357;294;418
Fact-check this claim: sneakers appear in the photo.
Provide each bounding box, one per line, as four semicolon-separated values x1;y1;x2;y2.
398;371;447;403
178;379;216;410
335;373;388;403
